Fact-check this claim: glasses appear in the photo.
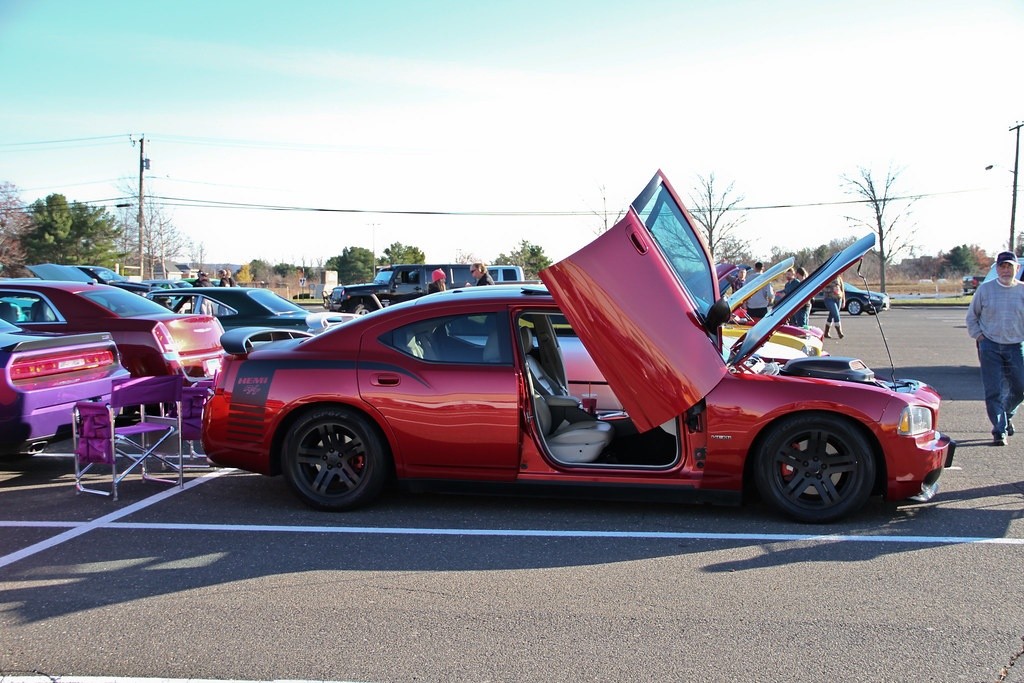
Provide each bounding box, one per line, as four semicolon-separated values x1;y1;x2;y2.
470;268;478;274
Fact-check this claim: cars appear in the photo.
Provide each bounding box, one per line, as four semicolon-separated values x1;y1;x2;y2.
0;263;347;346
0;277;227;423
0;314;132;463
451;256;831;411
774;277;891;316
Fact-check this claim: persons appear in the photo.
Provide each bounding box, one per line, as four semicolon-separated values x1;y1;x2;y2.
972;277;978;289
965;252;1024;446
821;273;845;338
218;269;236;315
785;267;814;327
428;268;447;294
721;258;747;309
193;270;216;316
742;262;774;318
465;262;495;287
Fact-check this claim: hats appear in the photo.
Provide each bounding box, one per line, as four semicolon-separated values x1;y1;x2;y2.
432;268;446;283
997;251;1017;265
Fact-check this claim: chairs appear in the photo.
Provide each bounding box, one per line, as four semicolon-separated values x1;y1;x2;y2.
0;302;24;321
70;375;215;500
30;301;44;321
401;271;410;283
484;327;637;462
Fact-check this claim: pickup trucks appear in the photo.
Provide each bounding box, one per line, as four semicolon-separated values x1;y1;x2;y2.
962;275;987;295
483;265;543;285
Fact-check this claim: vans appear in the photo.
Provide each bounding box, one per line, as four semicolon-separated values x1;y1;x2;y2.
973;257;1024;297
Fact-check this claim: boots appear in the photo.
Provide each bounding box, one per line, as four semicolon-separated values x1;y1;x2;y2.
824;324;831;338
835;325;844;339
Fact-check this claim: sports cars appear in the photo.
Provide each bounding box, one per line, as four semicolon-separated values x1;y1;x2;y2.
200;168;957;527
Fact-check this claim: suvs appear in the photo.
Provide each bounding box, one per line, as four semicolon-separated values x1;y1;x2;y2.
319;262;479;315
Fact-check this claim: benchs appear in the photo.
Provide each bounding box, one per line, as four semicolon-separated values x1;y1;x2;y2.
403;333;437;361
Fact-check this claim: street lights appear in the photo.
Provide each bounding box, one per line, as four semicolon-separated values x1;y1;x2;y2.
115;202;146;281
364;222;382;278
984;163;1020;251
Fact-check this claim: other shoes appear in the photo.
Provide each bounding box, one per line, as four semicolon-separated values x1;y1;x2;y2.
1006;420;1015;436
994;433;1007;445
